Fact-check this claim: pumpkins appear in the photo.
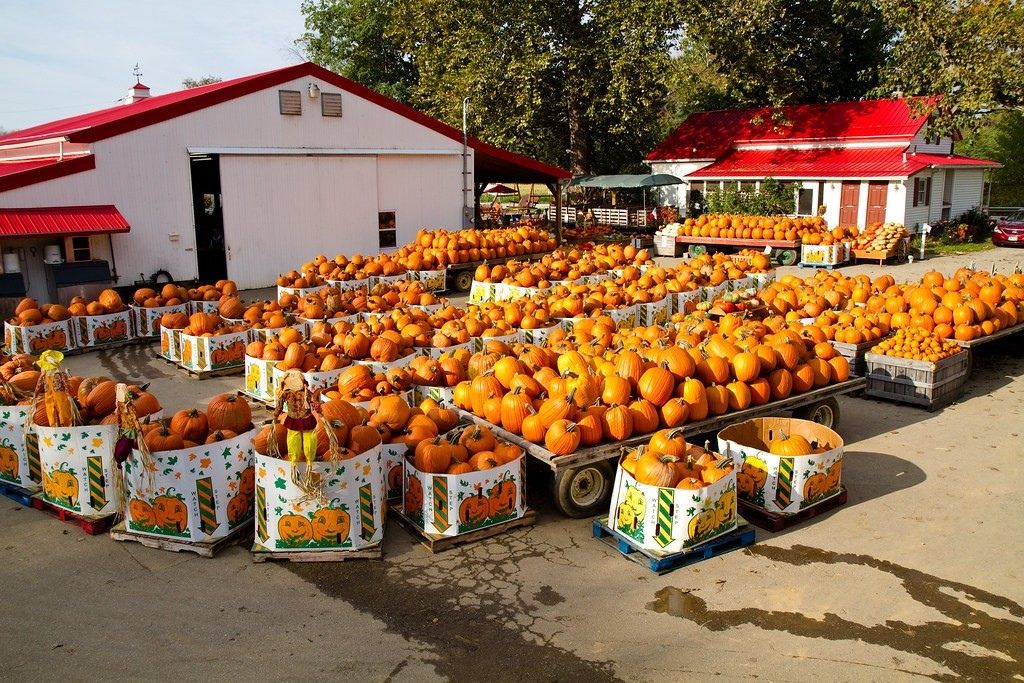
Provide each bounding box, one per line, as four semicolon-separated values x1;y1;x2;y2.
0;206;1024;490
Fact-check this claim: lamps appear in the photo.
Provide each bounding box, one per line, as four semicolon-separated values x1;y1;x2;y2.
308;82;320;98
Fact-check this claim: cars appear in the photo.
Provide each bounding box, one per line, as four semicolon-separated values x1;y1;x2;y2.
992;210;1024;247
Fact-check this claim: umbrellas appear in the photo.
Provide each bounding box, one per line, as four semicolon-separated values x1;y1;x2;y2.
482;185;519;194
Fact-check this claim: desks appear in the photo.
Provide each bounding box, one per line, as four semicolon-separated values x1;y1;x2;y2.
940;325;1023;383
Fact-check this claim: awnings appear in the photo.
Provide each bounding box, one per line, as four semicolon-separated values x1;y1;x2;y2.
0;204;131;238
564;173;688;188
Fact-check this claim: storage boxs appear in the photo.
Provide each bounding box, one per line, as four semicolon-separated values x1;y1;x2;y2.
0;183;973;562
